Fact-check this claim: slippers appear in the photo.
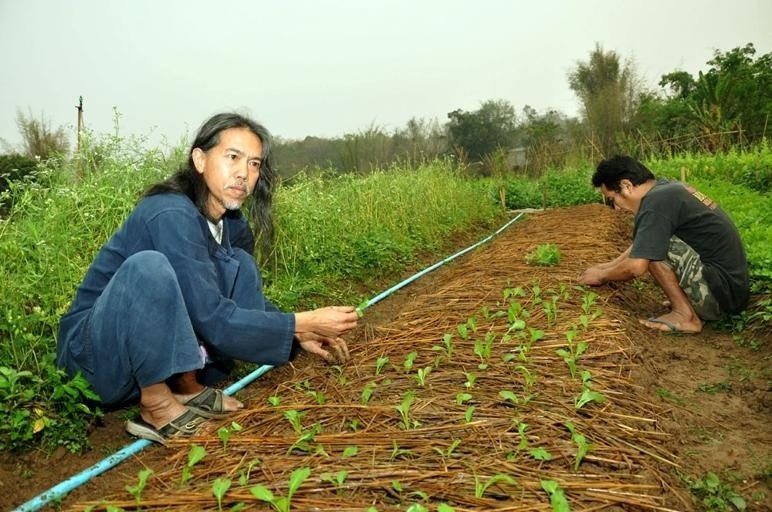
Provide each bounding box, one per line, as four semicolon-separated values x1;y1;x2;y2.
184;386;243;419
124;408;208;446
640;318;695;337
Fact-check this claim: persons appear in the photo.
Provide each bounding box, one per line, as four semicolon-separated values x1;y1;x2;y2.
57;114;358;446
578;155;749;334
